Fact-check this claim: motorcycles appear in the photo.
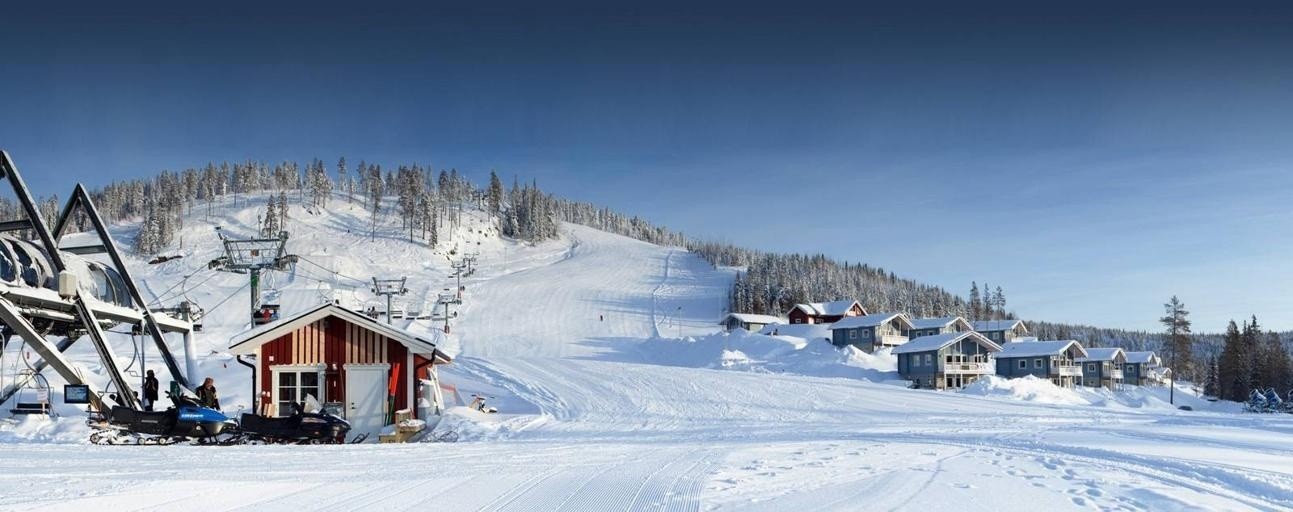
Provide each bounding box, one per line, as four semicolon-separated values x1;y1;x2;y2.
86;383;245;445
241;394;370;445
471;394;497;414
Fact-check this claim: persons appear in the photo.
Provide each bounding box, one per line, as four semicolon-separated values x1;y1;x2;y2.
141;369;158;411
110;392;141;406
254;307;280;325
194;377;220;411
